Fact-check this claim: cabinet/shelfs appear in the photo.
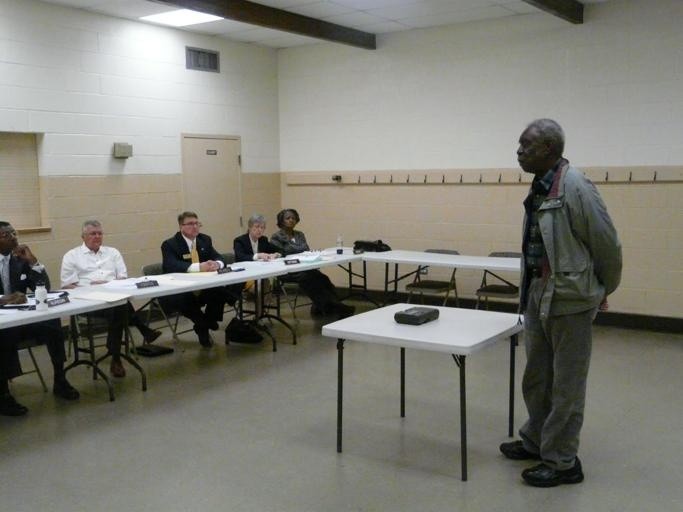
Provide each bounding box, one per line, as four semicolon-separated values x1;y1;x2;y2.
34;279;49;311
335;230;344;254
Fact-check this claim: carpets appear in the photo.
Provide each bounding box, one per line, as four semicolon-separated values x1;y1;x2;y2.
192;243;201;296
3;257;12;296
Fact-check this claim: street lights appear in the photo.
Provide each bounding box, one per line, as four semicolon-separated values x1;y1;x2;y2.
225;318;266;344
352;240;391;255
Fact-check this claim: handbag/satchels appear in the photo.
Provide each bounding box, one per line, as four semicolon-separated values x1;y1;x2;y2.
29;259;39;268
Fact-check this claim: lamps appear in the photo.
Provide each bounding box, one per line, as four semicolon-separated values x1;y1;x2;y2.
475;252;522;314
282;280;311;322
220;253;273;326
142;263;198;352
67;312;139;368
11;338;48;391
405;250;460;308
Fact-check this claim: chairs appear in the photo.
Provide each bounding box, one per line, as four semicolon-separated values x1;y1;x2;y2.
500;441;536;459
521;458;583;486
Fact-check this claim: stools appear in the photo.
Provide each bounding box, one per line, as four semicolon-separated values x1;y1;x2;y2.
530;270;542;278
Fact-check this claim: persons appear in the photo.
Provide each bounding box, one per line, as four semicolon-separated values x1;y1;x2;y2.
0;222;80;417
60;220;162;378
234;214;286;293
161;212;227;348
500;118;622;487
270;209;355;320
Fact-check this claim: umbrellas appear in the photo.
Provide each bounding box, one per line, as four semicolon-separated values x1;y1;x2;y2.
194;327;213;347
54;382;80;400
111;360;125;377
3;397;28;415
146;330;161;344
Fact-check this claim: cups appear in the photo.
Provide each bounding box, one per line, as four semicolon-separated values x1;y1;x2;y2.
0;230;18;240
182;222;201;226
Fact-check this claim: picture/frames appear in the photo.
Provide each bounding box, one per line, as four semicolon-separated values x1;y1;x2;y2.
363;249;523;315
81;261;288;390
321;303;524;481
231;248;365;344
1;291;127;401
